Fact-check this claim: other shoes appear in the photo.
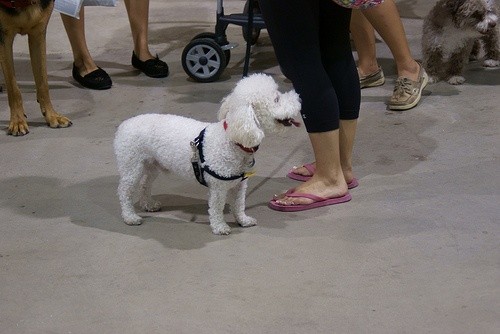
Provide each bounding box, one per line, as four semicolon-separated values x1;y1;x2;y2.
131;50;168;78
72;61;112;90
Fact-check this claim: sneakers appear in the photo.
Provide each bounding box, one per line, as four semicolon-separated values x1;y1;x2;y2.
357;64;384;88
388;62;429;109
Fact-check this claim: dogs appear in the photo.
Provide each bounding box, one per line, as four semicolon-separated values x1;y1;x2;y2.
113;73;301;235
0;0;73;136
420;0;500;86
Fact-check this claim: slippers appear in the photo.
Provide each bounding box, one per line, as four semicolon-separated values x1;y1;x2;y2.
287;164;359;189
268;187;352;212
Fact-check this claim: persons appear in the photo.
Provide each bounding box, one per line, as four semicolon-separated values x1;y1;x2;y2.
258;0;361;212
60;0;169;89
352;0;429;109
